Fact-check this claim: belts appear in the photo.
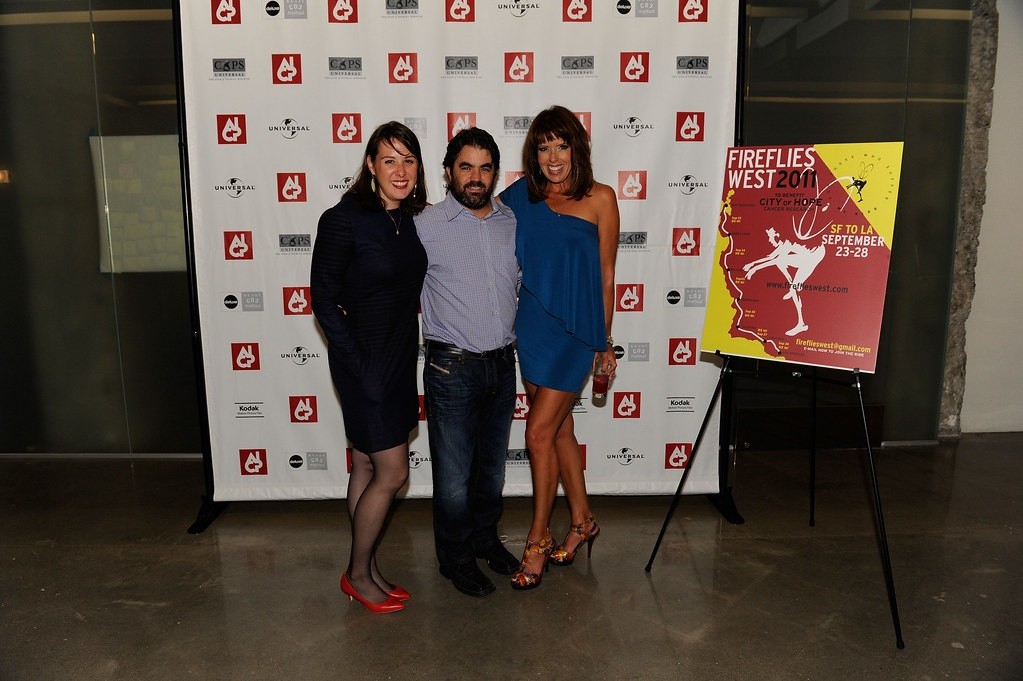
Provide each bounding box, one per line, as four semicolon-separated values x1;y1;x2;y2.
424;339;515;360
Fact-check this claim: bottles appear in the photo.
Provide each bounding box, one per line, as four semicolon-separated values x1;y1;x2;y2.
593;360;609;407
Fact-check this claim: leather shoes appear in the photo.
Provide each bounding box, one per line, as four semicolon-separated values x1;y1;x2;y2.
439;560;496;597
475;542;522;575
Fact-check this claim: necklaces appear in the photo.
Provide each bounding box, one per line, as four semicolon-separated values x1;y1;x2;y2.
386;210;401;234
556;209;560;217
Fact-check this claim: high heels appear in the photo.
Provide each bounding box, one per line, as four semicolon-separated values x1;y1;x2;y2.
386;584;411;601
550;514;600;566
340;571;404;614
511;526;556;590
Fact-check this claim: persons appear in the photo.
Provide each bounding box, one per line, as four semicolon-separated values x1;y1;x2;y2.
497;104;620;590
311;121;432;614
414;126;522;597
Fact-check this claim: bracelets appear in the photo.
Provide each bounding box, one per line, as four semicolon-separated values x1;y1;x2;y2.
606;336;613;345
